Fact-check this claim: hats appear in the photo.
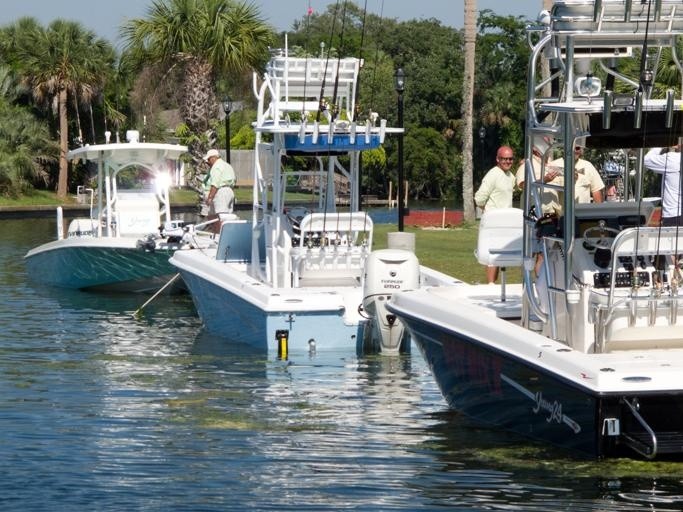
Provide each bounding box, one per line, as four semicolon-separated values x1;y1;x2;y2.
202;149;218;160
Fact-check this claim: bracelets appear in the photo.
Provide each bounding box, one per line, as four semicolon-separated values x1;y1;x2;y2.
208;197;212;200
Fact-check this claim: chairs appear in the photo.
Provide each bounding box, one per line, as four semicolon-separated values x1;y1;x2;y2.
291;211;373;291
474;206;525;300
589;223;683;359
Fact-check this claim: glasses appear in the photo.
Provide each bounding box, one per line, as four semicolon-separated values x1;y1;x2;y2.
500;158;512;160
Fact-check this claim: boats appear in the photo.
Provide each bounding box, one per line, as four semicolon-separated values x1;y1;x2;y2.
411;415;683;512
167;1;468;359
23;74;219;297
384;0;683;459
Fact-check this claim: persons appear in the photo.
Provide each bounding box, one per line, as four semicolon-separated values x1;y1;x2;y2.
473;143;518;286
203;148;236;232
548;138;602;203
642;136;681;268
515;128;563;277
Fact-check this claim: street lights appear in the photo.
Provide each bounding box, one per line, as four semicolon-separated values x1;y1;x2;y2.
392;67;408;232
477;125;486;177
220;93;234;188
